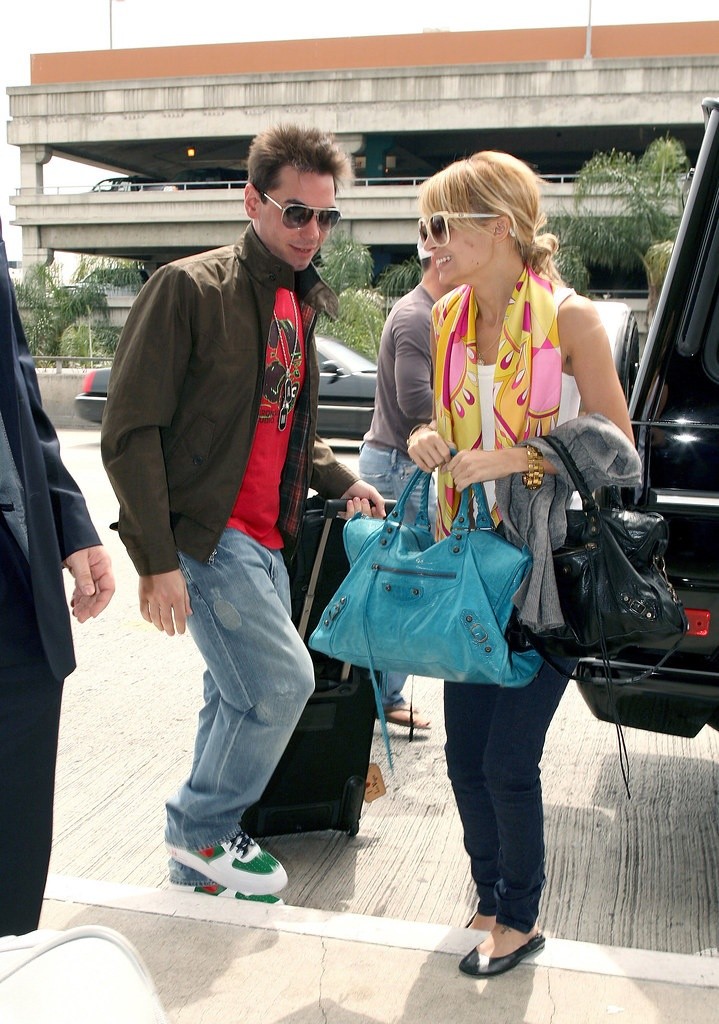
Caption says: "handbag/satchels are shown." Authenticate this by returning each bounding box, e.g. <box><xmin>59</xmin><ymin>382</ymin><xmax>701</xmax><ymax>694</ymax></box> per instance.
<box><xmin>308</xmin><ymin>447</ymin><xmax>542</xmax><ymax>690</ymax></box>
<box><xmin>507</xmin><ymin>433</ymin><xmax>688</xmax><ymax>685</ymax></box>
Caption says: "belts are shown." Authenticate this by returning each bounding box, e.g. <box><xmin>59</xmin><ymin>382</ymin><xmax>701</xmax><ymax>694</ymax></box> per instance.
<box><xmin>366</xmin><ymin>439</ymin><xmax>403</xmax><ymax>455</ymax></box>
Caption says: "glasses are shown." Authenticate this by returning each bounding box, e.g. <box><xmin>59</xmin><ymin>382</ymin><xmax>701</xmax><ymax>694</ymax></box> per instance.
<box><xmin>418</xmin><ymin>211</ymin><xmax>518</xmax><ymax>248</ymax></box>
<box><xmin>255</xmin><ymin>188</ymin><xmax>342</xmax><ymax>231</ymax></box>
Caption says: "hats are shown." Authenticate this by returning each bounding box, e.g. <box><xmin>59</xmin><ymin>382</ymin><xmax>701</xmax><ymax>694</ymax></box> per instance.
<box><xmin>417</xmin><ymin>240</ymin><xmax>432</xmax><ymax>259</ymax></box>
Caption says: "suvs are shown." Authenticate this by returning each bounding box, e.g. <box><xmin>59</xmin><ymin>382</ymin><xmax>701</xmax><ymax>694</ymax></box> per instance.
<box><xmin>533</xmin><ymin>100</ymin><xmax>719</xmax><ymax>741</ymax></box>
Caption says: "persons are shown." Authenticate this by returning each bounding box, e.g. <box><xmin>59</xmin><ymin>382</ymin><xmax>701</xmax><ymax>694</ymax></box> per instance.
<box><xmin>0</xmin><ymin>239</ymin><xmax>115</xmax><ymax>936</ymax></box>
<box><xmin>407</xmin><ymin>151</ymin><xmax>634</xmax><ymax>977</ymax></box>
<box><xmin>358</xmin><ymin>239</ymin><xmax>457</xmax><ymax>730</ymax></box>
<box><xmin>101</xmin><ymin>129</ymin><xmax>388</xmax><ymax>909</ymax></box>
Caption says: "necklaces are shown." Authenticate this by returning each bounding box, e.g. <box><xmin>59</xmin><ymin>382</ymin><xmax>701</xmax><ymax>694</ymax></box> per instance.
<box><xmin>476</xmin><ymin>334</ymin><xmax>500</xmax><ymax>365</ymax></box>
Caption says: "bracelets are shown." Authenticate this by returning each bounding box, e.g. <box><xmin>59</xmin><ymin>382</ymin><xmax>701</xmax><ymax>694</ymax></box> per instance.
<box><xmin>522</xmin><ymin>445</ymin><xmax>544</xmax><ymax>489</ymax></box>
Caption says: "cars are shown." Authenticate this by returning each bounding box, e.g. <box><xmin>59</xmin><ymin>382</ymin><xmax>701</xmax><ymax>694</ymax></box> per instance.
<box><xmin>75</xmin><ymin>333</ymin><xmax>378</xmax><ymax>442</ymax></box>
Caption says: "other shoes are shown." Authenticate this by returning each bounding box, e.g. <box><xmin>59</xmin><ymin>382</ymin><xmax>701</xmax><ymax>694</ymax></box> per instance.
<box><xmin>459</xmin><ymin>933</ymin><xmax>547</xmax><ymax>977</ymax></box>
<box><xmin>462</xmin><ymin>912</ymin><xmax>477</xmax><ymax>929</ymax></box>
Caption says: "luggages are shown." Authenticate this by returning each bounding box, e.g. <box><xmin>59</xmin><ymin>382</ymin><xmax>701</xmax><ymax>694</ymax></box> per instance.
<box><xmin>240</xmin><ymin>498</ymin><xmax>398</xmax><ymax>836</ymax></box>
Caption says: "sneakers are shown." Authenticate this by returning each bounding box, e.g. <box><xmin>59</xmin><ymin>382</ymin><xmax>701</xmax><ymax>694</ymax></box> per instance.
<box><xmin>168</xmin><ymin>833</ymin><xmax>288</xmax><ymax>895</ymax></box>
<box><xmin>192</xmin><ymin>886</ymin><xmax>284</xmax><ymax>905</ymax></box>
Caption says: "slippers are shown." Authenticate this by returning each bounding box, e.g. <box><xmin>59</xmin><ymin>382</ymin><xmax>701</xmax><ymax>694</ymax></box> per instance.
<box><xmin>378</xmin><ymin>699</ymin><xmax>432</xmax><ymax>730</ymax></box>
